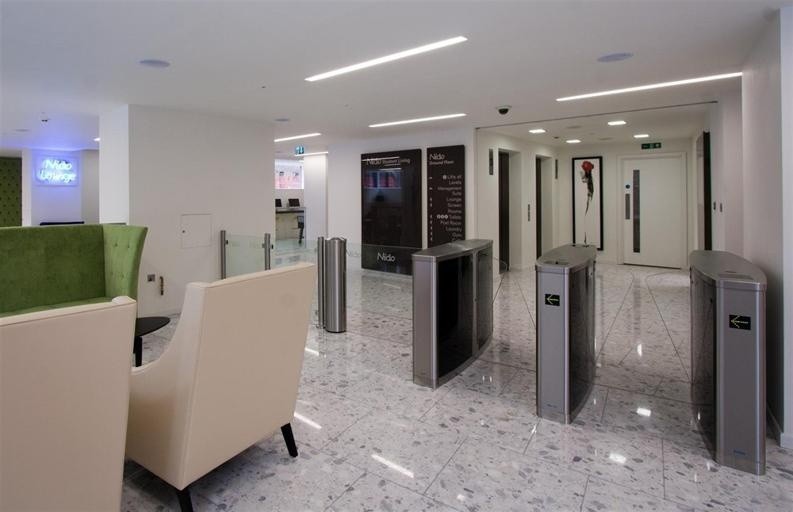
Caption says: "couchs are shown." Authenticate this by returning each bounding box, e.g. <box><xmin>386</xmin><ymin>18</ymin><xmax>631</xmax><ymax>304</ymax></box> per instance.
<box><xmin>0</xmin><ymin>222</ymin><xmax>148</xmax><ymax>318</ymax></box>
<box><xmin>0</xmin><ymin>295</ymin><xmax>138</xmax><ymax>507</ymax></box>
<box><xmin>125</xmin><ymin>261</ymin><xmax>317</xmax><ymax>511</ymax></box>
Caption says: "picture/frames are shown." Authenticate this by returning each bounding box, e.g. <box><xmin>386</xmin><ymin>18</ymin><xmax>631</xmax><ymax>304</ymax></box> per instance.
<box><xmin>571</xmin><ymin>155</ymin><xmax>603</xmax><ymax>251</ymax></box>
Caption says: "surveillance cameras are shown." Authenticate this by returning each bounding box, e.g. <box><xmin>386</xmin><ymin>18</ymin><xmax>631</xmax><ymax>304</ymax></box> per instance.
<box><xmin>497</xmin><ymin>105</ymin><xmax>512</xmax><ymax>114</ymax></box>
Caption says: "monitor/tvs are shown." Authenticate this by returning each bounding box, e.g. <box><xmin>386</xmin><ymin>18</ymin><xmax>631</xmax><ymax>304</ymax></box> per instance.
<box><xmin>363</xmin><ymin>167</ymin><xmax>407</xmax><ymax>208</ymax></box>
<box><xmin>275</xmin><ymin>200</ymin><xmax>282</xmax><ymax>207</ymax></box>
<box><xmin>288</xmin><ymin>199</ymin><xmax>299</xmax><ymax>208</ymax></box>
